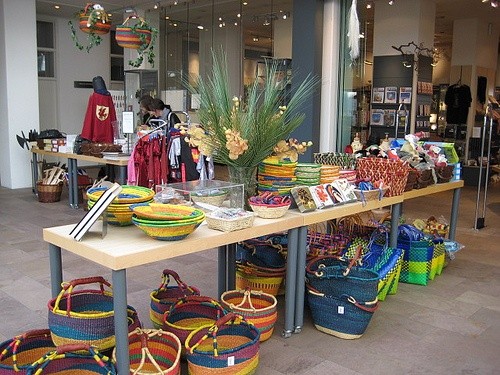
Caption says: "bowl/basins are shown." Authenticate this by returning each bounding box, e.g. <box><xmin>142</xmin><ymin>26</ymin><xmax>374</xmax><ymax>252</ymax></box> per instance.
<box><xmin>86</xmin><ymin>185</ymin><xmax>155</xmax><ymax>227</ymax></box>
<box><xmin>247</xmin><ymin>200</ymin><xmax>292</xmax><ymax>219</ymax></box>
<box><xmin>257</xmin><ymin>161</ymin><xmax>297</xmax><ymax>195</ymax></box>
<box><xmin>129</xmin><ymin>203</ymin><xmax>206</xmax><ymax>242</ymax></box>
<box><xmin>295</xmin><ymin>162</ymin><xmax>357</xmax><ymax>188</ymax></box>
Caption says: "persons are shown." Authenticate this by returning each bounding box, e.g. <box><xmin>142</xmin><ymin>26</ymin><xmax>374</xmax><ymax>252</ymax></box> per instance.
<box><xmin>134</xmin><ymin>89</ymin><xmax>200</xmax><ymax>183</ymax></box>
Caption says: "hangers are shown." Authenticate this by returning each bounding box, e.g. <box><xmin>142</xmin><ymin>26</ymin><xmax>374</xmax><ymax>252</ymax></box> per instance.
<box><xmin>452</xmin><ymin>83</ymin><xmax>462</xmax><ymax>86</ymax></box>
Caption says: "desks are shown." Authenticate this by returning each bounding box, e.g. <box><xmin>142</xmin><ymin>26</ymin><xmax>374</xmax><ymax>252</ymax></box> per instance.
<box><xmin>228</xmin><ymin>195</ymin><xmax>403</xmax><ymax>333</ymax></box>
<box><xmin>43</xmin><ymin>212</ymin><xmax>304</xmax><ymax>375</ymax></box>
<box><xmin>31</xmin><ymin>148</ymin><xmax>131</xmax><ymax>208</ymax></box>
<box><xmin>332</xmin><ymin>180</ymin><xmax>464</xmax><ymax>242</ymax></box>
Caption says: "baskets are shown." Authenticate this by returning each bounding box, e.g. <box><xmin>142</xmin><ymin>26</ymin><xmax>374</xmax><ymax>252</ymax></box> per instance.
<box><xmin>115</xmin><ymin>15</ymin><xmax>152</xmax><ymax>50</ymax></box>
<box><xmin>206</xmin><ymin>209</ymin><xmax>252</xmax><ymax>231</ymax></box>
<box><xmin>248</xmin><ymin>195</ymin><xmax>290</xmax><ymax>218</ymax></box>
<box><xmin>1</xmin><ymin>269</ymin><xmax>278</xmax><ymax>375</ymax></box>
<box><xmin>79</xmin><ymin>3</ymin><xmax>111</xmax><ymax>35</ymax></box>
<box><xmin>37</xmin><ymin>180</ymin><xmax>61</xmax><ymax>202</ymax></box>
<box><xmin>84</xmin><ymin>143</ymin><xmax>122</xmax><ymax>156</ymax></box>
<box><xmin>235</xmin><ymin>210</ymin><xmax>443</xmax><ymax>340</ymax></box>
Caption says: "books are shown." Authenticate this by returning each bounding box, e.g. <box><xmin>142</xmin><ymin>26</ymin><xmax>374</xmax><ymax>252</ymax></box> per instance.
<box><xmin>68</xmin><ymin>183</ymin><xmax>122</xmax><ymax>242</ymax></box>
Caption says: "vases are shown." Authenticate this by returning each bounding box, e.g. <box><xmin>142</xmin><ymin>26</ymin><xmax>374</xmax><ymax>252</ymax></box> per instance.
<box><xmin>228</xmin><ymin>166</ymin><xmax>258</xmax><ymax>211</ymax></box>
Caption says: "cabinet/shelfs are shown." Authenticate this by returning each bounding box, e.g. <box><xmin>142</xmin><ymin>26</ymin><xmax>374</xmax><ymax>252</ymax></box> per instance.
<box><xmin>371</xmin><ymin>103</ymin><xmax>410</xmax><ymax>130</ymax></box>
<box><xmin>416</xmin><ymin>93</ymin><xmax>433</xmax><ymax>129</ymax></box>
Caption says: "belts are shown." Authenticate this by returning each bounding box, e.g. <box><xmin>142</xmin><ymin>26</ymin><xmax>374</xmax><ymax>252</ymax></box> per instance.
<box><xmin>360</xmin><ymin>156</ymin><xmax>439</xmax><ymax>196</ymax></box>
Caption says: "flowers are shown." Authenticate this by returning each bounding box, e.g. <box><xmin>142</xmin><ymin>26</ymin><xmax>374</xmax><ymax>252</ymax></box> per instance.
<box><xmin>178</xmin><ymin>44</ymin><xmax>322</xmax><ymax>166</ymax></box>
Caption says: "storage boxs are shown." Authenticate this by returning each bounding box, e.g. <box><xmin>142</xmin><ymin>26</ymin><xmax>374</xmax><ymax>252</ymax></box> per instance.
<box><xmin>156</xmin><ymin>179</ymin><xmax>244</xmax><ymax>210</ymax></box>
